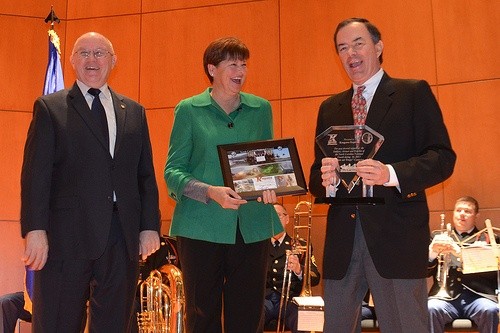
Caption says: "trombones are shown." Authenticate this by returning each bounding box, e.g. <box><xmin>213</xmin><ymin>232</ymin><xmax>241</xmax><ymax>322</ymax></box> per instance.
<box><xmin>277</xmin><ymin>192</ymin><xmax>313</xmax><ymax>333</ymax></box>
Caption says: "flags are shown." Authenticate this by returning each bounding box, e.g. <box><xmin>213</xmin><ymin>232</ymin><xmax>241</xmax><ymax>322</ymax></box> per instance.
<box><xmin>24</xmin><ymin>33</ymin><xmax>64</xmax><ymax>303</ymax></box>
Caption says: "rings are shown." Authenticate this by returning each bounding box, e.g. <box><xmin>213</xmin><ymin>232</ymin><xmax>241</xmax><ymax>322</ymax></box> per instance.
<box><xmin>153</xmin><ymin>248</ymin><xmax>156</xmax><ymax>251</ymax></box>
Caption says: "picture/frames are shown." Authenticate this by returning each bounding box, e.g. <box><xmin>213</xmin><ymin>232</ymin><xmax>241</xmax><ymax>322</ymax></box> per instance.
<box><xmin>217</xmin><ymin>137</ymin><xmax>308</xmax><ymax>200</ymax></box>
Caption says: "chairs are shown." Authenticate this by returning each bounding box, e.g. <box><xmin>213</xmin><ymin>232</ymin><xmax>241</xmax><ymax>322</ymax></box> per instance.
<box><xmin>360</xmin><ymin>291</ymin><xmax>499</xmax><ymax>333</ymax></box>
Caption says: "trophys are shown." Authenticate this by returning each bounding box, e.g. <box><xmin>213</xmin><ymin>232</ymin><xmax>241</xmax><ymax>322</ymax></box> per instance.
<box><xmin>313</xmin><ymin>125</ymin><xmax>385</xmax><ymax>205</ymax></box>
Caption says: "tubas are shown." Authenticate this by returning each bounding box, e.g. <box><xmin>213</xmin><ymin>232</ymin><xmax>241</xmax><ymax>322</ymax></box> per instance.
<box><xmin>136</xmin><ymin>264</ymin><xmax>188</xmax><ymax>333</ymax></box>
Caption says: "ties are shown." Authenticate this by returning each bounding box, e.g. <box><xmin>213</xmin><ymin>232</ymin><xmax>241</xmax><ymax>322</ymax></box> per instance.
<box><xmin>87</xmin><ymin>87</ymin><xmax>111</xmax><ymax>150</ymax></box>
<box><xmin>274</xmin><ymin>241</ymin><xmax>281</xmax><ymax>250</ymax></box>
<box><xmin>460</xmin><ymin>233</ymin><xmax>471</xmax><ymax>246</ymax></box>
<box><xmin>350</xmin><ymin>85</ymin><xmax>371</xmax><ymax>144</ymax></box>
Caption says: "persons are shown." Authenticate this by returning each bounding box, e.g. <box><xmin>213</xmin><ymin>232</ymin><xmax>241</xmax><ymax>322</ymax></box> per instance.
<box><xmin>163</xmin><ymin>37</ymin><xmax>284</xmax><ymax>333</ymax></box>
<box><xmin>427</xmin><ymin>196</ymin><xmax>500</xmax><ymax>333</ymax></box>
<box><xmin>264</xmin><ymin>202</ymin><xmax>321</xmax><ymax>333</ymax></box>
<box><xmin>309</xmin><ymin>18</ymin><xmax>456</xmax><ymax>333</ymax></box>
<box><xmin>19</xmin><ymin>31</ymin><xmax>162</xmax><ymax>333</ymax></box>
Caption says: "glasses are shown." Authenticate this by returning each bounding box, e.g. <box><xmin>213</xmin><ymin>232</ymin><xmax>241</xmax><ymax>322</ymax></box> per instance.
<box><xmin>73</xmin><ymin>48</ymin><xmax>114</xmax><ymax>59</ymax></box>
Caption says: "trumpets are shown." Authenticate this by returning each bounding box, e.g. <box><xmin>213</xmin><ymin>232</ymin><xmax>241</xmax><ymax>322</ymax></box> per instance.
<box><xmin>435</xmin><ymin>213</ymin><xmax>452</xmax><ymax>299</ymax></box>
